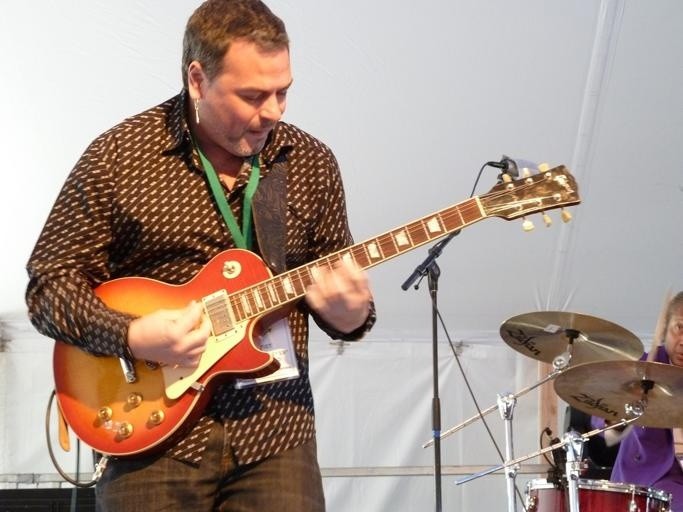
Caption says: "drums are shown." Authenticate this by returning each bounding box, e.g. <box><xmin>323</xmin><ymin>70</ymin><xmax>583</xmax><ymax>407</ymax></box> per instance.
<box><xmin>524</xmin><ymin>479</ymin><xmax>674</xmax><ymax>512</ymax></box>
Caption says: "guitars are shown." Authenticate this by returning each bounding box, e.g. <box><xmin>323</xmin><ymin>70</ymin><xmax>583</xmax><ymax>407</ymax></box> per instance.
<box><xmin>53</xmin><ymin>162</ymin><xmax>581</xmax><ymax>457</ymax></box>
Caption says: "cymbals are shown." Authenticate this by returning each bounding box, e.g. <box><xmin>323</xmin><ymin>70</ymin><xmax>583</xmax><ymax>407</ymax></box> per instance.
<box><xmin>500</xmin><ymin>311</ymin><xmax>644</xmax><ymax>366</ymax></box>
<box><xmin>554</xmin><ymin>359</ymin><xmax>683</xmax><ymax>428</ymax></box>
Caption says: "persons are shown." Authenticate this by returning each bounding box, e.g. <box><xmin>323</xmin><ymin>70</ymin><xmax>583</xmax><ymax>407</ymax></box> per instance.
<box><xmin>23</xmin><ymin>2</ymin><xmax>379</xmax><ymax>512</ymax></box>
<box><xmin>589</xmin><ymin>290</ymin><xmax>682</xmax><ymax>511</ymax></box>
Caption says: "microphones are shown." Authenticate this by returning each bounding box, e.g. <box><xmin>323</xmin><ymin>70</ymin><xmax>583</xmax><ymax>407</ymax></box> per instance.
<box><xmin>546</xmin><ymin>428</ymin><xmax>567</xmax><ymax>462</ymax></box>
<box><xmin>487</xmin><ymin>155</ymin><xmax>549</xmax><ymax>181</ymax></box>
